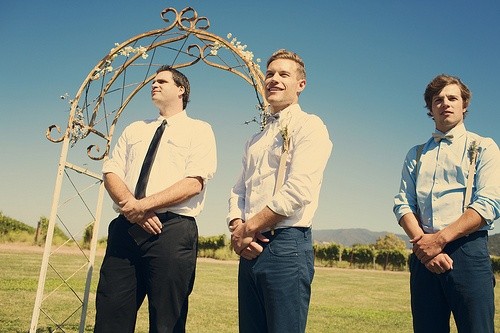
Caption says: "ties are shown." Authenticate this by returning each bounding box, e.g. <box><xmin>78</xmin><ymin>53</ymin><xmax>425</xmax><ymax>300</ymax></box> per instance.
<box><xmin>135</xmin><ymin>120</ymin><xmax>167</xmax><ymax>200</ymax></box>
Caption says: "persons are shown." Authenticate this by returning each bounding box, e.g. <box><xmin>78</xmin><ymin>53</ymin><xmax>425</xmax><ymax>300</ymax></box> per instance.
<box><xmin>392</xmin><ymin>80</ymin><xmax>500</xmax><ymax>333</ymax></box>
<box><xmin>226</xmin><ymin>47</ymin><xmax>333</xmax><ymax>333</ymax></box>
<box><xmin>93</xmin><ymin>64</ymin><xmax>217</xmax><ymax>333</ymax></box>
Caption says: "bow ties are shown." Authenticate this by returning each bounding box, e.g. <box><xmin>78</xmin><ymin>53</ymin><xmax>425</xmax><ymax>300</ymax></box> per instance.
<box><xmin>266</xmin><ymin>112</ymin><xmax>282</xmax><ymax>122</ymax></box>
<box><xmin>432</xmin><ymin>132</ymin><xmax>454</xmax><ymax>144</ymax></box>
